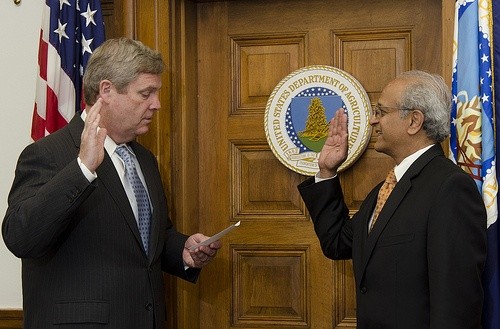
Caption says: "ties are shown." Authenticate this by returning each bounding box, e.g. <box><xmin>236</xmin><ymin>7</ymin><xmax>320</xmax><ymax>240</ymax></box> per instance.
<box><xmin>115</xmin><ymin>146</ymin><xmax>151</xmax><ymax>256</ymax></box>
<box><xmin>370</xmin><ymin>169</ymin><xmax>398</xmax><ymax>232</ymax></box>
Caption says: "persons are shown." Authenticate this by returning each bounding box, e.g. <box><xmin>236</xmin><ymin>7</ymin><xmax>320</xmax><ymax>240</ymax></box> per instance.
<box><xmin>297</xmin><ymin>70</ymin><xmax>488</xmax><ymax>329</ymax></box>
<box><xmin>1</xmin><ymin>38</ymin><xmax>222</xmax><ymax>329</ymax></box>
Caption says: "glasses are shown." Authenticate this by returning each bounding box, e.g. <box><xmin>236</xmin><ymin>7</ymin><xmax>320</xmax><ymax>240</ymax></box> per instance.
<box><xmin>372</xmin><ymin>105</ymin><xmax>426</xmax><ymax>122</ymax></box>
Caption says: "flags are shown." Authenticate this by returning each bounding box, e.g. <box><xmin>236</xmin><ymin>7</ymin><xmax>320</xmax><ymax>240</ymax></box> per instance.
<box><xmin>31</xmin><ymin>0</ymin><xmax>106</xmax><ymax>141</ymax></box>
<box><xmin>449</xmin><ymin>0</ymin><xmax>500</xmax><ymax>329</ymax></box>
